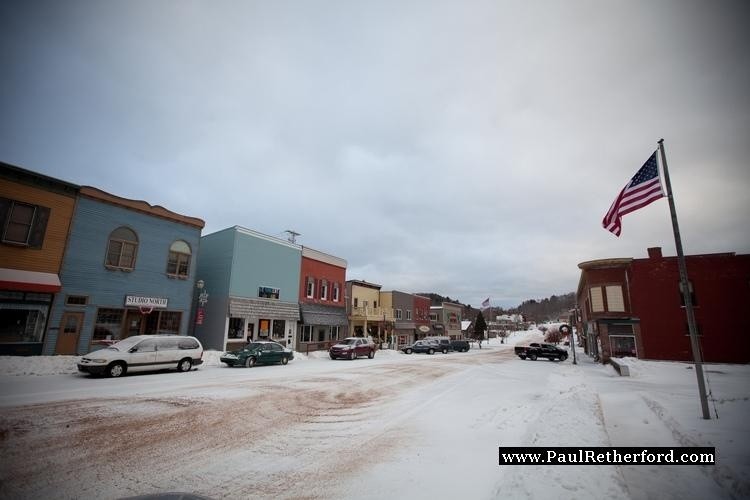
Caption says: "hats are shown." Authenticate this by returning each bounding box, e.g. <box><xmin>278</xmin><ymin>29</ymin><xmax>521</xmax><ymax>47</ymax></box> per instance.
<box><xmin>451</xmin><ymin>340</ymin><xmax>470</xmax><ymax>352</ymax></box>
<box><xmin>401</xmin><ymin>340</ymin><xmax>439</xmax><ymax>354</ymax></box>
<box><xmin>219</xmin><ymin>341</ymin><xmax>294</xmax><ymax>368</ymax></box>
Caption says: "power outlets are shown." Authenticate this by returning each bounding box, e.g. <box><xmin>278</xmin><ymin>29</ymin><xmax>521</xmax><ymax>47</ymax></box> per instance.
<box><xmin>514</xmin><ymin>342</ymin><xmax>568</xmax><ymax>361</ymax></box>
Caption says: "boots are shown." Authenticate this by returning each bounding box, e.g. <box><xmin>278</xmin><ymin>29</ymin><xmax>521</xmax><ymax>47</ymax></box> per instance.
<box><xmin>329</xmin><ymin>337</ymin><xmax>378</xmax><ymax>360</ymax></box>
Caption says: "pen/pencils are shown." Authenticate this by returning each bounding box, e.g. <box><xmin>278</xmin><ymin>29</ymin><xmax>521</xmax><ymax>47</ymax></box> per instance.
<box><xmin>423</xmin><ymin>336</ymin><xmax>454</xmax><ymax>354</ymax></box>
<box><xmin>77</xmin><ymin>334</ymin><xmax>204</xmax><ymax>379</ymax></box>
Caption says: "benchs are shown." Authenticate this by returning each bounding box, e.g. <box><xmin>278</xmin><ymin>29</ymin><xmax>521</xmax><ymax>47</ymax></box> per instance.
<box><xmin>482</xmin><ymin>298</ymin><xmax>489</xmax><ymax>307</ymax></box>
<box><xmin>601</xmin><ymin>150</ymin><xmax>666</xmax><ymax>238</ymax></box>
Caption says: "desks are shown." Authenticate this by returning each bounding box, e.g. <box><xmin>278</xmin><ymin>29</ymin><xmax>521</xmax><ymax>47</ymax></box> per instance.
<box><xmin>192</xmin><ymin>278</ymin><xmax>206</xmax><ymax>335</ymax></box>
